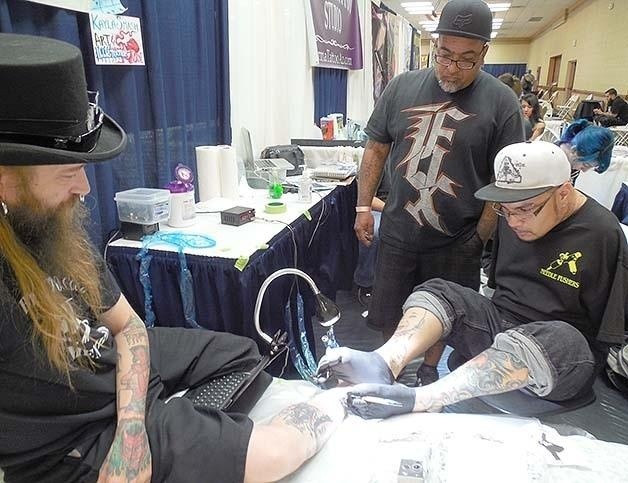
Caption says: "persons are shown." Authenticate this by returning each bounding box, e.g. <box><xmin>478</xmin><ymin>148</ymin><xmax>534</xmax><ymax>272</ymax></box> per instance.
<box><xmin>496</xmin><ymin>68</ymin><xmax>628</xmax><ymax>188</ymax></box>
<box><xmin>352</xmin><ymin>0</ymin><xmax>527</xmax><ymax>388</ymax></box>
<box><xmin>309</xmin><ymin>139</ymin><xmax>628</xmax><ymax>423</ymax></box>
<box><xmin>353</xmin><ymin>156</ymin><xmax>392</xmax><ymax>307</ymax></box>
<box><xmin>0</xmin><ymin>30</ymin><xmax>348</xmax><ymax>482</ymax></box>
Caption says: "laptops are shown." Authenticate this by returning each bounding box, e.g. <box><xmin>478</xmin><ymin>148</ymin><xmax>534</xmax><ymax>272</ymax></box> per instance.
<box><xmin>181</xmin><ymin>354</ymin><xmax>271</xmax><ymax>413</ymax></box>
<box><xmin>241</xmin><ymin>126</ymin><xmax>296</xmax><ymax>171</ymax></box>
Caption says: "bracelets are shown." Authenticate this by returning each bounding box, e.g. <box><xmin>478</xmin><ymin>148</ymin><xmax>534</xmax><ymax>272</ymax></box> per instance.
<box><xmin>355</xmin><ymin>206</ymin><xmax>372</xmax><ymax>213</ymax></box>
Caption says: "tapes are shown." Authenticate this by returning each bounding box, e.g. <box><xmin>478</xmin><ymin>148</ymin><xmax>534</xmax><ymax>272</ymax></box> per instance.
<box><xmin>265</xmin><ymin>201</ymin><xmax>286</xmax><ymax>213</ymax></box>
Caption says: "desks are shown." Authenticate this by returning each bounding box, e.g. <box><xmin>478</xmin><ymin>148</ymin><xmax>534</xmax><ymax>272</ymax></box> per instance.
<box><xmin>108</xmin><ymin>134</ymin><xmax>367</xmax><ymax>377</ymax></box>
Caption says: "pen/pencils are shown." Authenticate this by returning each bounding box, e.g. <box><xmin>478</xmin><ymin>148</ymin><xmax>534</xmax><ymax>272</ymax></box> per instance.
<box><xmin>351</xmin><ymin>394</ymin><xmax>404</xmax><ymax>409</ymax></box>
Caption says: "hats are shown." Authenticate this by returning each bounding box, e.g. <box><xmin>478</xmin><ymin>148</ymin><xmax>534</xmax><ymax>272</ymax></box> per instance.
<box><xmin>1</xmin><ymin>32</ymin><xmax>128</xmax><ymax>166</ymax></box>
<box><xmin>430</xmin><ymin>0</ymin><xmax>493</xmax><ymax>42</ymax></box>
<box><xmin>475</xmin><ymin>140</ymin><xmax>571</xmax><ymax>204</ymax></box>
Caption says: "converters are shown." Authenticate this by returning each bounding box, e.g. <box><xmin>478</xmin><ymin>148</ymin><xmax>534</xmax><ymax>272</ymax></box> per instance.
<box><xmin>220</xmin><ymin>206</ymin><xmax>256</xmax><ymax>226</ymax></box>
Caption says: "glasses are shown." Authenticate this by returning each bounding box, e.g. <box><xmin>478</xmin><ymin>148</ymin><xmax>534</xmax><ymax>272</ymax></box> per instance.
<box><xmin>433</xmin><ymin>38</ymin><xmax>485</xmax><ymax>70</ymax></box>
<box><xmin>491</xmin><ymin>187</ymin><xmax>560</xmax><ymax>222</ymax></box>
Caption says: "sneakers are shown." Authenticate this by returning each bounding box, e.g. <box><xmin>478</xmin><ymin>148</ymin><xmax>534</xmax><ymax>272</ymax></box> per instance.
<box><xmin>415</xmin><ymin>372</ymin><xmax>442</xmax><ymax>413</ymax></box>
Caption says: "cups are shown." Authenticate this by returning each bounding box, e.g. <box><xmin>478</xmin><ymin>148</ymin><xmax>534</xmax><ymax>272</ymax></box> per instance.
<box><xmin>320</xmin><ymin>118</ymin><xmax>334</xmax><ymax>140</ymax></box>
<box><xmin>269</xmin><ymin>168</ymin><xmax>284</xmax><ymax>199</ymax></box>
<box><xmin>298</xmin><ymin>178</ymin><xmax>313</xmax><ymax>203</ymax></box>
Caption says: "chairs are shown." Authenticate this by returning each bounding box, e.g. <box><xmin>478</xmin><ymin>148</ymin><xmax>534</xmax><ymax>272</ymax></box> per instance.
<box><xmin>535</xmin><ymin>90</ymin><xmax>592</xmax><ymax>138</ymax></box>
<box><xmin>606</xmin><ymin>124</ymin><xmax>628</xmax><ymax>147</ymax></box>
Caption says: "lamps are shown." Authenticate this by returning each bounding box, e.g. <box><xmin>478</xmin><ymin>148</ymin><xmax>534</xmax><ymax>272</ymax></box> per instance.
<box><xmin>253</xmin><ymin>267</ymin><xmax>340</xmax><ymax>353</ymax></box>
